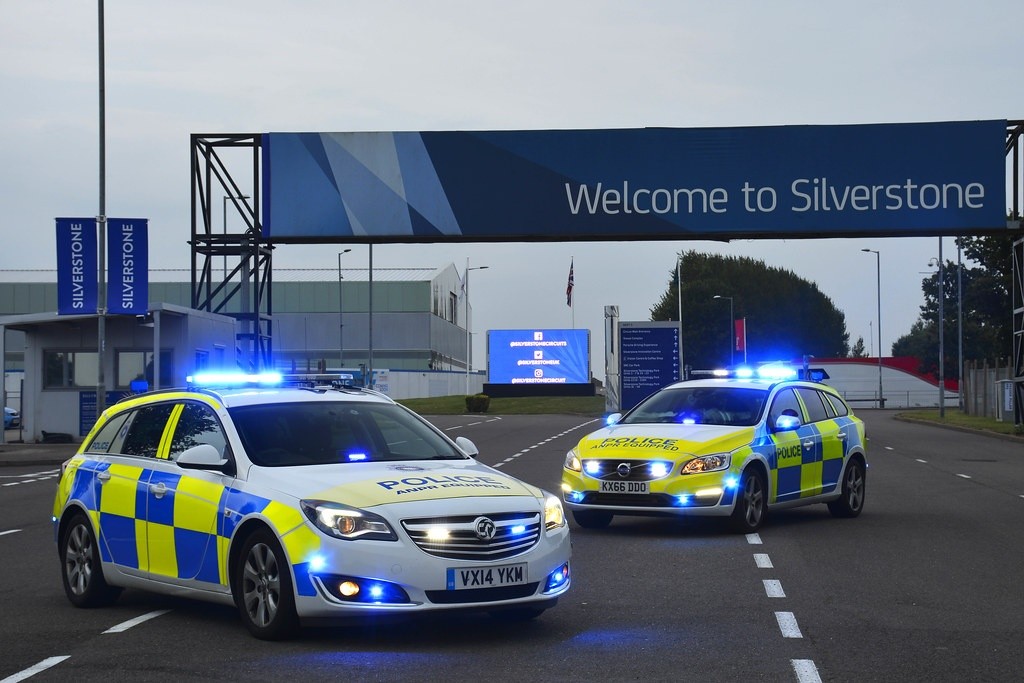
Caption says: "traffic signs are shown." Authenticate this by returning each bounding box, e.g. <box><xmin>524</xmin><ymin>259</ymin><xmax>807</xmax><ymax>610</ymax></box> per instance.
<box><xmin>617</xmin><ymin>321</ymin><xmax>683</xmax><ymax>411</ymax></box>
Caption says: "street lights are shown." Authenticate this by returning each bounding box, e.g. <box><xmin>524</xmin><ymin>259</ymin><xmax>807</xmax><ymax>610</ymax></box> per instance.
<box><xmin>713</xmin><ymin>296</ymin><xmax>734</xmax><ymax>370</ymax></box>
<box><xmin>223</xmin><ymin>195</ymin><xmax>252</xmax><ymax>313</ymax></box>
<box><xmin>860</xmin><ymin>248</ymin><xmax>885</xmax><ymax>408</ymax></box>
<box><xmin>466</xmin><ymin>266</ymin><xmax>491</xmax><ymax>396</ymax></box>
<box><xmin>927</xmin><ymin>236</ymin><xmax>944</xmax><ymax>415</ymax></box>
<box><xmin>337</xmin><ymin>249</ymin><xmax>353</xmax><ymax>368</ymax></box>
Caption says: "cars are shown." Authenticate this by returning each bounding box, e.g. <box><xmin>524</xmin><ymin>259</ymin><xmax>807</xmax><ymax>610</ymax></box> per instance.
<box><xmin>562</xmin><ymin>363</ymin><xmax>869</xmax><ymax>534</ymax></box>
<box><xmin>53</xmin><ymin>371</ymin><xmax>574</xmax><ymax>641</ymax></box>
<box><xmin>4</xmin><ymin>407</ymin><xmax>20</xmax><ymax>428</ymax></box>
